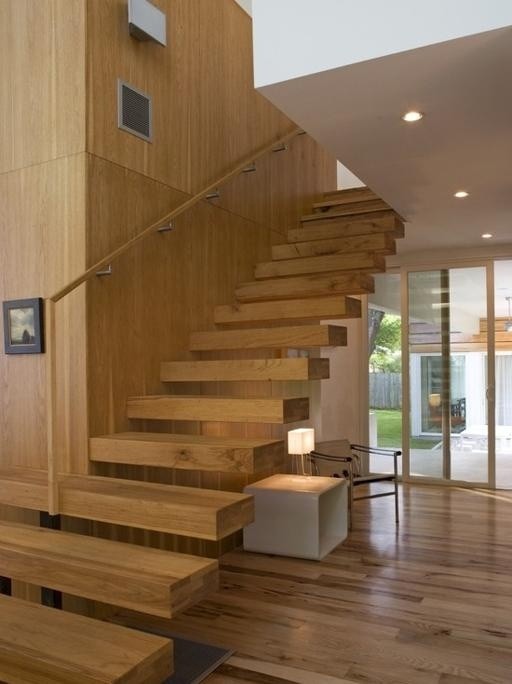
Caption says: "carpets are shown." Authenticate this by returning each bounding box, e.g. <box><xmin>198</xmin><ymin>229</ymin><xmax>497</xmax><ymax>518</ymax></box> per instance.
<box><xmin>126</xmin><ymin>623</ymin><xmax>239</xmax><ymax>684</ymax></box>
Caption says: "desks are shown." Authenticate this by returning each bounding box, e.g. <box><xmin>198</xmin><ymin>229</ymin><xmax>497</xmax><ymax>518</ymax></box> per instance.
<box><xmin>243</xmin><ymin>473</ymin><xmax>349</xmax><ymax>561</ymax></box>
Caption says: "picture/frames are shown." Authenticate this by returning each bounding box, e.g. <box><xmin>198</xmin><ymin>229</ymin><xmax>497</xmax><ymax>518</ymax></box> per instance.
<box><xmin>2</xmin><ymin>296</ymin><xmax>44</xmax><ymax>354</ymax></box>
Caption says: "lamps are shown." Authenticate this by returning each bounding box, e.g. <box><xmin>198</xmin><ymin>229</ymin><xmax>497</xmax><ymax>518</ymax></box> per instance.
<box><xmin>287</xmin><ymin>427</ymin><xmax>316</xmax><ymax>476</ymax></box>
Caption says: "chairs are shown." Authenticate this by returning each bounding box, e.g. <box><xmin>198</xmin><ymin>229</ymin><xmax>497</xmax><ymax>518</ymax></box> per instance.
<box><xmin>305</xmin><ymin>439</ymin><xmax>402</xmax><ymax>530</ymax></box>
